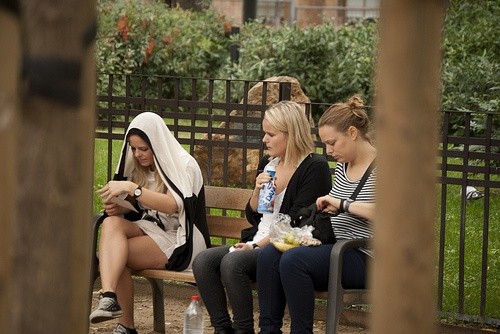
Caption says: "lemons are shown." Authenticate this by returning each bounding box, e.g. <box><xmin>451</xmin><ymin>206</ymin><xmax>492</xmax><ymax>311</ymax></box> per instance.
<box><xmin>283</xmin><ymin>235</ymin><xmax>300</xmax><ymax>246</ymax></box>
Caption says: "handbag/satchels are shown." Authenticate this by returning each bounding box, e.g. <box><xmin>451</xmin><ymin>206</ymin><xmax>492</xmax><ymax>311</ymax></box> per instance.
<box><xmin>290</xmin><ymin>203</ymin><xmax>337</xmax><ymax>245</ymax></box>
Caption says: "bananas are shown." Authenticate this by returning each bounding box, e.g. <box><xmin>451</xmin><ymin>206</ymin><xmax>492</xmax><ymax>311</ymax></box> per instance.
<box><xmin>273</xmin><ymin>241</ymin><xmax>297</xmax><ymax>251</ymax></box>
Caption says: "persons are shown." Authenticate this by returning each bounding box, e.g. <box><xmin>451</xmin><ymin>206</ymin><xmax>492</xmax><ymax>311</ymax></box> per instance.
<box><xmin>256</xmin><ymin>95</ymin><xmax>376</xmax><ymax>334</ymax></box>
<box><xmin>89</xmin><ymin>112</ymin><xmax>211</xmax><ymax>334</ymax></box>
<box><xmin>192</xmin><ymin>100</ymin><xmax>333</xmax><ymax>334</ymax></box>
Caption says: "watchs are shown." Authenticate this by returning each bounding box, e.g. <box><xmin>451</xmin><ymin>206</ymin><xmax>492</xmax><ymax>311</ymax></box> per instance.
<box><xmin>252</xmin><ymin>244</ymin><xmax>260</xmax><ymax>249</ymax></box>
<box><xmin>344</xmin><ymin>199</ymin><xmax>354</xmax><ymax>213</ymax></box>
<box><xmin>132</xmin><ymin>186</ymin><xmax>142</xmax><ymax>198</ymax></box>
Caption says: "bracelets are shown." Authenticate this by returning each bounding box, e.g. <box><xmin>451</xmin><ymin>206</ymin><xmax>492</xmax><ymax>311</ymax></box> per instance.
<box><xmin>340</xmin><ymin>198</ymin><xmax>347</xmax><ymax>213</ymax></box>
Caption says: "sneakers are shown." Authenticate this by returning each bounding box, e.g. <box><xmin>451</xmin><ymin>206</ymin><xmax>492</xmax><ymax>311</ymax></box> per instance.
<box><xmin>89</xmin><ymin>289</ymin><xmax>123</xmax><ymax>323</ymax></box>
<box><xmin>110</xmin><ymin>323</ymin><xmax>138</xmax><ymax>334</ymax></box>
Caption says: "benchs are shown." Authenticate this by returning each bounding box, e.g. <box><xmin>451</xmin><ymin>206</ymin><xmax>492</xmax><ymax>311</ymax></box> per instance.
<box><xmin>93</xmin><ymin>187</ymin><xmax>372</xmax><ymax>334</ymax></box>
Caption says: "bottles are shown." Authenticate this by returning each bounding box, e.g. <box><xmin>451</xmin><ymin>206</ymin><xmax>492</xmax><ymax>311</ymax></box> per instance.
<box><xmin>257</xmin><ymin>165</ymin><xmax>277</xmax><ymax>214</ymax></box>
<box><xmin>184</xmin><ymin>295</ymin><xmax>205</xmax><ymax>334</ymax></box>
